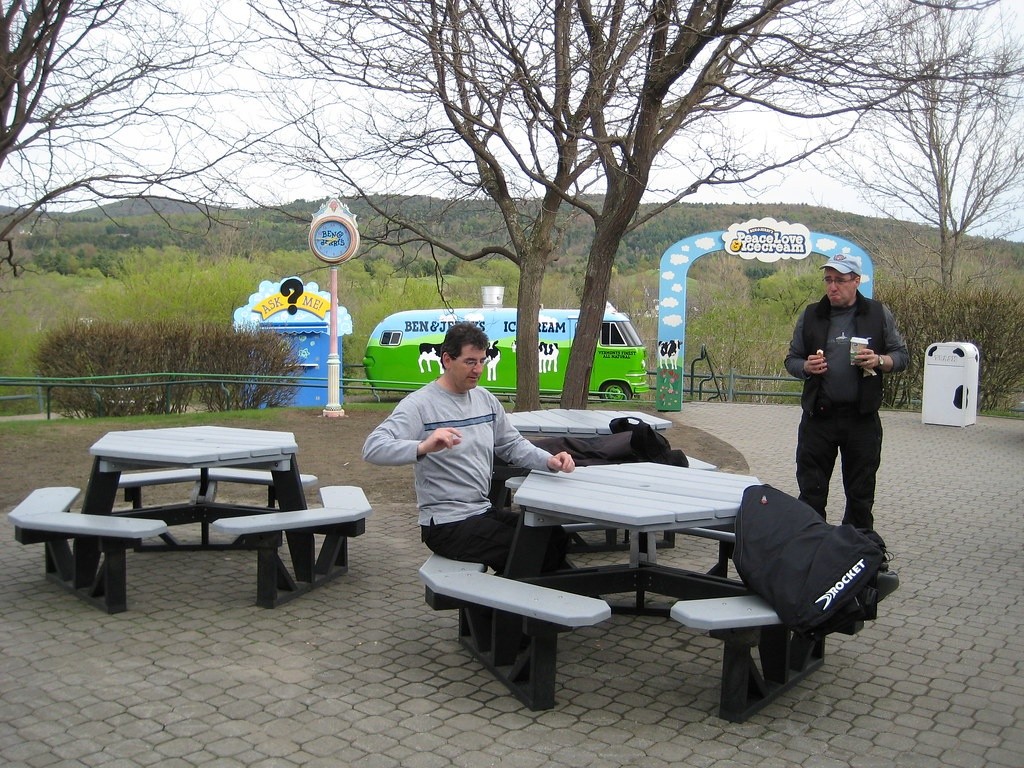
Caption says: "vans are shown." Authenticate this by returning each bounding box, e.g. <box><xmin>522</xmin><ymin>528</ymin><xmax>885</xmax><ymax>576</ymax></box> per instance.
<box><xmin>362</xmin><ymin>308</ymin><xmax>650</xmax><ymax>404</ymax></box>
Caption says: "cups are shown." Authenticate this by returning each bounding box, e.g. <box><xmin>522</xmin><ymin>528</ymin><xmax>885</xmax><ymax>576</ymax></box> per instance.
<box><xmin>850</xmin><ymin>337</ymin><xmax>869</xmax><ymax>365</ymax></box>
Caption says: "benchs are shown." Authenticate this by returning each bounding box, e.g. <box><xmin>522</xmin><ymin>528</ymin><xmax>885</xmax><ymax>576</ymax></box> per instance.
<box><xmin>7</xmin><ymin>408</ymin><xmax>902</xmax><ymax>725</ymax></box>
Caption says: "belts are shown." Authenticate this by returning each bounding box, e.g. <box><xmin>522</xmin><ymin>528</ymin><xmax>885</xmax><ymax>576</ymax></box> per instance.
<box><xmin>816</xmin><ymin>406</ymin><xmax>848</xmax><ymax>413</ymax></box>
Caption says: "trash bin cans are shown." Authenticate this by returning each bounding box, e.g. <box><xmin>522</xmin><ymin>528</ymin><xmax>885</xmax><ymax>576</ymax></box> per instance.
<box><xmin>919</xmin><ymin>341</ymin><xmax>981</xmax><ymax>426</ymax></box>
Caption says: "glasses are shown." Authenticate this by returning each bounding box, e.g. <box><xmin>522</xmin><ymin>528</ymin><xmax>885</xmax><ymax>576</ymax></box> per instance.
<box><xmin>453</xmin><ymin>356</ymin><xmax>487</xmax><ymax>368</ymax></box>
<box><xmin>822</xmin><ymin>276</ymin><xmax>854</xmax><ymax>285</ymax></box>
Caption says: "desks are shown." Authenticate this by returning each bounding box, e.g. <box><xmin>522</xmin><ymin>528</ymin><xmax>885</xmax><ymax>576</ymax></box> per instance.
<box><xmin>70</xmin><ymin>425</ymin><xmax>317</xmax><ymax>586</ymax></box>
<box><xmin>492</xmin><ymin>462</ymin><xmax>792</xmax><ymax>687</ymax></box>
<box><xmin>504</xmin><ymin>409</ymin><xmax>673</xmax><ymax>439</ymax></box>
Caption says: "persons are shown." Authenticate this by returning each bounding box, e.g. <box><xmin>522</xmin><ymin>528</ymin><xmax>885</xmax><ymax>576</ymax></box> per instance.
<box><xmin>362</xmin><ymin>321</ymin><xmax>575</xmax><ymax>632</ymax></box>
<box><xmin>784</xmin><ymin>253</ymin><xmax>910</xmax><ymax>571</ymax></box>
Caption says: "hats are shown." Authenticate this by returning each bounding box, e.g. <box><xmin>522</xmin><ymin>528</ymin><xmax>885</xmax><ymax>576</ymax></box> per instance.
<box><xmin>819</xmin><ymin>253</ymin><xmax>861</xmax><ymax>276</ymax></box>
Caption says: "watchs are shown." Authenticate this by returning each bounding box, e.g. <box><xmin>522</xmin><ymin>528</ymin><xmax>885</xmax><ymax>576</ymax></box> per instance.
<box><xmin>876</xmin><ymin>354</ymin><xmax>884</xmax><ymax>367</ymax></box>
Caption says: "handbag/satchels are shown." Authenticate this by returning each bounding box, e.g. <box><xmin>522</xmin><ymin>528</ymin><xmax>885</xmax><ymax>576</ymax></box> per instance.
<box><xmin>609</xmin><ymin>416</ymin><xmax>671</xmax><ymax>458</ymax></box>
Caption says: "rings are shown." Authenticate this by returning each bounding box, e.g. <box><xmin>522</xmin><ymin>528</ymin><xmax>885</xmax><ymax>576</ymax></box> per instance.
<box><xmin>867</xmin><ymin>361</ymin><xmax>869</xmax><ymax>365</ymax></box>
